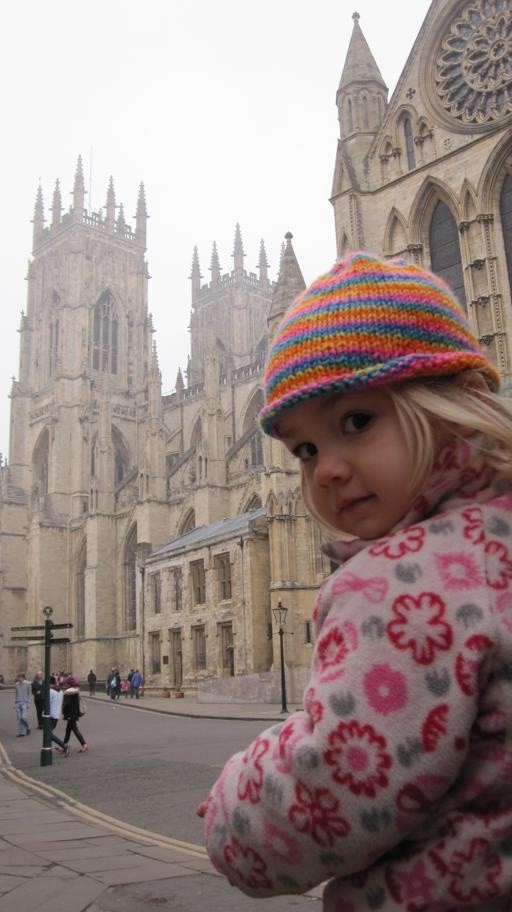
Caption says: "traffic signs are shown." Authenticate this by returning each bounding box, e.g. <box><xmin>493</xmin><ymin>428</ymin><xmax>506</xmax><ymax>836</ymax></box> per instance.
<box><xmin>10</xmin><ymin>625</ymin><xmax>44</xmax><ymax>632</ymax></box>
<box><xmin>50</xmin><ymin>638</ymin><xmax>71</xmax><ymax>644</ymax></box>
<box><xmin>10</xmin><ymin>636</ymin><xmax>45</xmax><ymax>642</ymax></box>
<box><xmin>50</xmin><ymin>622</ymin><xmax>76</xmax><ymax>630</ymax></box>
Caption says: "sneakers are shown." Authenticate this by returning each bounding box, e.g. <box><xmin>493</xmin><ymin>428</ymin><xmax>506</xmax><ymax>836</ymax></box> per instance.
<box><xmin>55</xmin><ymin>743</ymin><xmax>89</xmax><ymax>757</ymax></box>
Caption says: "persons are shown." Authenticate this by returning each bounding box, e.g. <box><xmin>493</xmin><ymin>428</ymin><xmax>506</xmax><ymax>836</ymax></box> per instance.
<box><xmin>13</xmin><ymin>667</ymin><xmax>143</xmax><ymax>757</ymax></box>
<box><xmin>196</xmin><ymin>251</ymin><xmax>511</xmax><ymax>911</ymax></box>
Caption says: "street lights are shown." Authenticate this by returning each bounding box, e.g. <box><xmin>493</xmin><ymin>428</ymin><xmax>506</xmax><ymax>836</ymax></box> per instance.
<box><xmin>271</xmin><ymin>597</ymin><xmax>290</xmax><ymax>715</ymax></box>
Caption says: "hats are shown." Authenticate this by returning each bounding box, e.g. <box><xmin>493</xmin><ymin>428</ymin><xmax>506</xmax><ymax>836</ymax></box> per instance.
<box><xmin>253</xmin><ymin>251</ymin><xmax>504</xmax><ymax>437</ymax></box>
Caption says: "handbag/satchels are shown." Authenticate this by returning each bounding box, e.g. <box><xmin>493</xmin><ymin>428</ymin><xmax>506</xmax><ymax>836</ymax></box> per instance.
<box><xmin>78</xmin><ymin>695</ymin><xmax>87</xmax><ymax>718</ymax></box>
